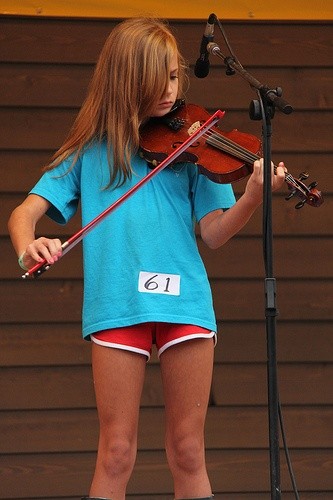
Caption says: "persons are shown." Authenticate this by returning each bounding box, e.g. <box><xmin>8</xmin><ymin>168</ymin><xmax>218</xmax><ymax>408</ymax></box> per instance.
<box><xmin>8</xmin><ymin>17</ymin><xmax>288</xmax><ymax>500</ymax></box>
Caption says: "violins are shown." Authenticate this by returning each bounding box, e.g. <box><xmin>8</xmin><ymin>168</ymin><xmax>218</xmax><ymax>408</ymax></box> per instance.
<box><xmin>136</xmin><ymin>103</ymin><xmax>323</xmax><ymax>212</ymax></box>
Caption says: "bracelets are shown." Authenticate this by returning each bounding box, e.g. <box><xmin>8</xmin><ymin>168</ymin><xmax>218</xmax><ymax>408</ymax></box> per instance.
<box><xmin>18</xmin><ymin>253</ymin><xmax>28</xmax><ymax>271</ymax></box>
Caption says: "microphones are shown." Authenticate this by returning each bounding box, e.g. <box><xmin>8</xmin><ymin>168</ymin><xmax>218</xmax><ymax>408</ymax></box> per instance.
<box><xmin>194</xmin><ymin>14</ymin><xmax>215</xmax><ymax>77</ymax></box>
